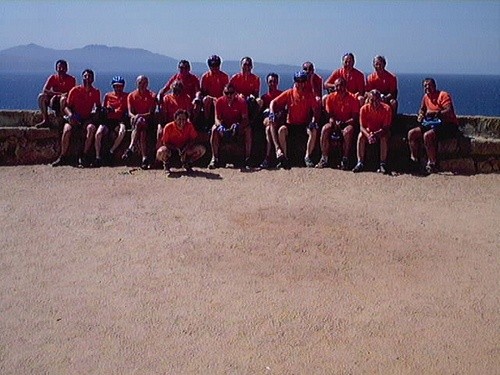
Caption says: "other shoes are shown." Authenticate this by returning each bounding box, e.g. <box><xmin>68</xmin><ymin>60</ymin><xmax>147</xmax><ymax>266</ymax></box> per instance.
<box><xmin>141</xmin><ymin>158</ymin><xmax>152</xmax><ymax>169</ymax></box>
<box><xmin>78</xmin><ymin>153</ymin><xmax>90</xmax><ymax>168</ymax></box>
<box><xmin>304</xmin><ymin>156</ymin><xmax>316</xmax><ymax>167</ymax></box>
<box><xmin>207</xmin><ymin>159</ymin><xmax>220</xmax><ymax>168</ymax></box>
<box><xmin>379</xmin><ymin>162</ymin><xmax>390</xmax><ymax>174</ymax></box>
<box><xmin>94</xmin><ymin>148</ymin><xmax>114</xmax><ymax>167</ymax></box>
<box><xmin>352</xmin><ymin>159</ymin><xmax>369</xmax><ymax>172</ymax></box>
<box><xmin>276</xmin><ymin>157</ymin><xmax>288</xmax><ymax>167</ymax></box>
<box><xmin>203</xmin><ymin>121</ymin><xmax>211</xmax><ymax>131</ymax></box>
<box><xmin>341</xmin><ymin>159</ymin><xmax>351</xmax><ymax>170</ymax></box>
<box><xmin>245</xmin><ymin>160</ymin><xmax>262</xmax><ymax>171</ymax></box>
<box><xmin>36</xmin><ymin>119</ymin><xmax>52</xmax><ymax>128</ymax></box>
<box><xmin>52</xmin><ymin>155</ymin><xmax>69</xmax><ymax>167</ymax></box>
<box><xmin>260</xmin><ymin>159</ymin><xmax>272</xmax><ymax>168</ymax></box>
<box><xmin>315</xmin><ymin>158</ymin><xmax>330</xmax><ymax>168</ymax></box>
<box><xmin>276</xmin><ymin>147</ymin><xmax>284</xmax><ymax>158</ymax></box>
<box><xmin>122</xmin><ymin>149</ymin><xmax>133</xmax><ymax>161</ymax></box>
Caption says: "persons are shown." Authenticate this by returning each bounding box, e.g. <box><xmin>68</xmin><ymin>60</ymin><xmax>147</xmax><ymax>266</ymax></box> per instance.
<box><xmin>89</xmin><ymin>75</ymin><xmax>130</xmax><ymax>167</ymax></box>
<box><xmin>403</xmin><ymin>77</ymin><xmax>459</xmax><ymax>177</ymax></box>
<box><xmin>35</xmin><ymin>59</ymin><xmax>76</xmax><ymax>129</ymax></box>
<box><xmin>155</xmin><ymin>53</ymin><xmax>400</xmax><ymax>174</ymax></box>
<box><xmin>52</xmin><ymin>69</ymin><xmax>101</xmax><ymax>168</ymax></box>
<box><xmin>121</xmin><ymin>74</ymin><xmax>157</xmax><ymax>170</ymax></box>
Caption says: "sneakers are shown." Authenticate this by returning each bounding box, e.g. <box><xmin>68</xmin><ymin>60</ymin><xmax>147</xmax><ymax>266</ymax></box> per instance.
<box><xmin>424</xmin><ymin>157</ymin><xmax>440</xmax><ymax>174</ymax></box>
<box><xmin>404</xmin><ymin>157</ymin><xmax>420</xmax><ymax>173</ymax></box>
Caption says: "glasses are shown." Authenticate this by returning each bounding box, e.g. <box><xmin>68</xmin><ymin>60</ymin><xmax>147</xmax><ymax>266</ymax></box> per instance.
<box><xmin>269</xmin><ymin>80</ymin><xmax>273</xmax><ymax>83</ymax></box>
<box><xmin>223</xmin><ymin>91</ymin><xmax>234</xmax><ymax>94</ymax></box>
<box><xmin>243</xmin><ymin>63</ymin><xmax>250</xmax><ymax>66</ymax></box>
<box><xmin>303</xmin><ymin>67</ymin><xmax>312</xmax><ymax>70</ymax></box>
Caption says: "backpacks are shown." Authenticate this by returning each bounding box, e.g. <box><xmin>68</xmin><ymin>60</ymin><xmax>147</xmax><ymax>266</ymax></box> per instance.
<box><xmin>420</xmin><ymin>114</ymin><xmax>445</xmax><ymax>132</ymax></box>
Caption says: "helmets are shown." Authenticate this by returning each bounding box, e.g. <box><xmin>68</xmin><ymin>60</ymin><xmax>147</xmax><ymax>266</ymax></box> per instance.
<box><xmin>208</xmin><ymin>55</ymin><xmax>220</xmax><ymax>67</ymax></box>
<box><xmin>295</xmin><ymin>70</ymin><xmax>307</xmax><ymax>82</ymax></box>
<box><xmin>112</xmin><ymin>76</ymin><xmax>125</xmax><ymax>86</ymax></box>
<box><xmin>330</xmin><ymin>134</ymin><xmax>343</xmax><ymax>146</ymax></box>
